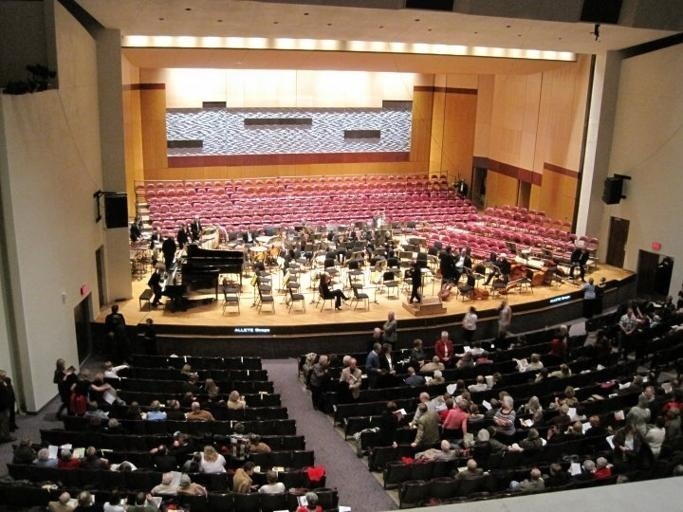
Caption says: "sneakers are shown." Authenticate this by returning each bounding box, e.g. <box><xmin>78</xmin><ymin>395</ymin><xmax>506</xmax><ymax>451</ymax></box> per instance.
<box><xmin>569</xmin><ymin>277</ymin><xmax>588</xmax><ymax>283</ymax></box>
<box><xmin>152</xmin><ymin>301</ymin><xmax>165</xmax><ymax>308</ymax></box>
<box><xmin>409</xmin><ymin>299</ymin><xmax>423</xmax><ymax>304</ymax></box>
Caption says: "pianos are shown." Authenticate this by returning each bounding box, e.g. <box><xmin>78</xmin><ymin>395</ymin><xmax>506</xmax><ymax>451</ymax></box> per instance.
<box><xmin>160</xmin><ymin>245</ymin><xmax>244</xmax><ymax>312</ymax></box>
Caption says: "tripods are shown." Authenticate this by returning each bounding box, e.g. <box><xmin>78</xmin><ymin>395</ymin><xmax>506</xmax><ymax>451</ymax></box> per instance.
<box><xmin>369</xmin><ymin>283</ymin><xmax>379</xmax><ymax>305</ymax></box>
<box><xmin>251</xmin><ymin>288</ymin><xmax>261</xmax><ymax>309</ymax></box>
<box><xmin>309</xmin><ymin>282</ymin><xmax>320</xmax><ymax>304</ymax></box>
<box><xmin>274</xmin><ymin>272</ymin><xmax>283</xmax><ymax>293</ymax></box>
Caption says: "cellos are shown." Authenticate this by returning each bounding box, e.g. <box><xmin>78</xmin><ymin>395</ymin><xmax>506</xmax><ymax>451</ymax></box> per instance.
<box><xmin>438</xmin><ymin>276</ymin><xmax>456</xmax><ymax>298</ymax></box>
<box><xmin>499</xmin><ymin>276</ymin><xmax>529</xmax><ymax>293</ymax></box>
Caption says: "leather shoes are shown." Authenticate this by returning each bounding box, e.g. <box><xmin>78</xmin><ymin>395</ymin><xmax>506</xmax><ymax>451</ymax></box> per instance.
<box><xmin>482</xmin><ymin>282</ymin><xmax>488</xmax><ymax>285</ymax></box>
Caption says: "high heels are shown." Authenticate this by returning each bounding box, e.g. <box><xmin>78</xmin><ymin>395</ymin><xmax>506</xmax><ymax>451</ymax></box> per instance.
<box><xmin>345</xmin><ymin>297</ymin><xmax>351</xmax><ymax>302</ymax></box>
<box><xmin>334</xmin><ymin>306</ymin><xmax>342</xmax><ymax>311</ymax></box>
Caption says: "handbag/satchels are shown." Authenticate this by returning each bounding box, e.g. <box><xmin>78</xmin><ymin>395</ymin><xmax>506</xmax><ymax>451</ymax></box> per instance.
<box><xmin>256</xmin><ymin>441</ymin><xmax>272</xmax><ymax>455</ymax></box>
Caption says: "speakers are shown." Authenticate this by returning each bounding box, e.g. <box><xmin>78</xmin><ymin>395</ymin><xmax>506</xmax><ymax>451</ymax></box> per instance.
<box><xmin>0</xmin><ymin>0</ymin><xmax>59</xmax><ymax>94</ymax></box>
<box><xmin>601</xmin><ymin>173</ymin><xmax>625</xmax><ymax>205</ymax></box>
<box><xmin>103</xmin><ymin>189</ymin><xmax>130</xmax><ymax>230</ymax></box>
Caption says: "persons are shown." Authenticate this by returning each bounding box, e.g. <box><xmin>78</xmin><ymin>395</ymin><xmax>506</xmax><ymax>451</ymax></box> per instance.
<box><xmin>451</xmin><ymin>178</ymin><xmax>468</xmax><ymax>199</ymax></box>
<box><xmin>301</xmin><ymin>292</ymin><xmax>682</xmax><ymax>511</ymax></box>
<box><xmin>127</xmin><ymin>207</ymin><xmax>594</xmax><ymax>313</ymax></box>
<box><xmin>1</xmin><ymin>304</ymin><xmax>323</xmax><ymax>512</ymax></box>
<box><xmin>594</xmin><ymin>276</ymin><xmax>607</xmax><ymax>313</ymax></box>
